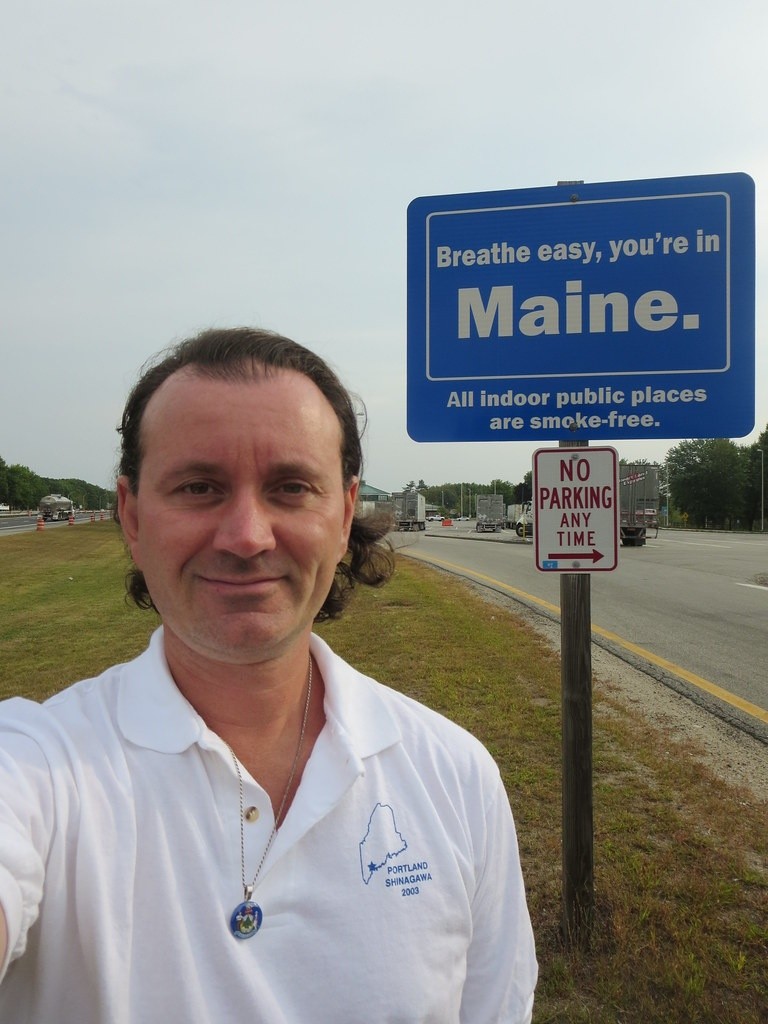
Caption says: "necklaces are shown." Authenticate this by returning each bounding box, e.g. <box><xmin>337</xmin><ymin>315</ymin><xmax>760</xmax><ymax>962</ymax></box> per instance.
<box><xmin>184</xmin><ymin>653</ymin><xmax>313</xmax><ymax>941</ymax></box>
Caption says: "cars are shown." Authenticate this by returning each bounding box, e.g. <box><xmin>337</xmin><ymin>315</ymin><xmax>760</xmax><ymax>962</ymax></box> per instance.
<box><xmin>457</xmin><ymin>516</ymin><xmax>470</xmax><ymax>521</ymax></box>
<box><xmin>0</xmin><ymin>503</ymin><xmax>9</xmax><ymax>511</ymax></box>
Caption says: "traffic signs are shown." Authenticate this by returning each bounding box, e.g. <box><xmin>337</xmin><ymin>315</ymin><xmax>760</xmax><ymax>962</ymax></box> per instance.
<box><xmin>407</xmin><ymin>171</ymin><xmax>757</xmax><ymax>570</ymax></box>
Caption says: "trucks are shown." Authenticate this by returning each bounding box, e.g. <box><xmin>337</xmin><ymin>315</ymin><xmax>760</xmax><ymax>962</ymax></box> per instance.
<box><xmin>392</xmin><ymin>492</ymin><xmax>426</xmax><ymax>532</ymax></box>
<box><xmin>37</xmin><ymin>494</ymin><xmax>76</xmax><ymax>521</ymax></box>
<box><xmin>474</xmin><ymin>494</ymin><xmax>503</xmax><ymax>534</ymax></box>
<box><xmin>619</xmin><ymin>463</ymin><xmax>660</xmax><ymax>547</ymax></box>
<box><xmin>506</xmin><ymin>504</ymin><xmax>523</xmax><ymax>529</ymax></box>
<box><xmin>516</xmin><ymin>498</ymin><xmax>533</xmax><ymax>537</ymax></box>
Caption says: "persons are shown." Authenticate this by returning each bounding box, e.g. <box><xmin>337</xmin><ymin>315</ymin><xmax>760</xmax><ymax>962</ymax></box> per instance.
<box><xmin>0</xmin><ymin>329</ymin><xmax>542</xmax><ymax>1024</ymax></box>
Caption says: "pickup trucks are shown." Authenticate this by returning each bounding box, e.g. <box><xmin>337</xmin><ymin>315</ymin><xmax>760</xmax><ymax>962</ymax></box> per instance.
<box><xmin>426</xmin><ymin>515</ymin><xmax>445</xmax><ymax>522</ymax></box>
